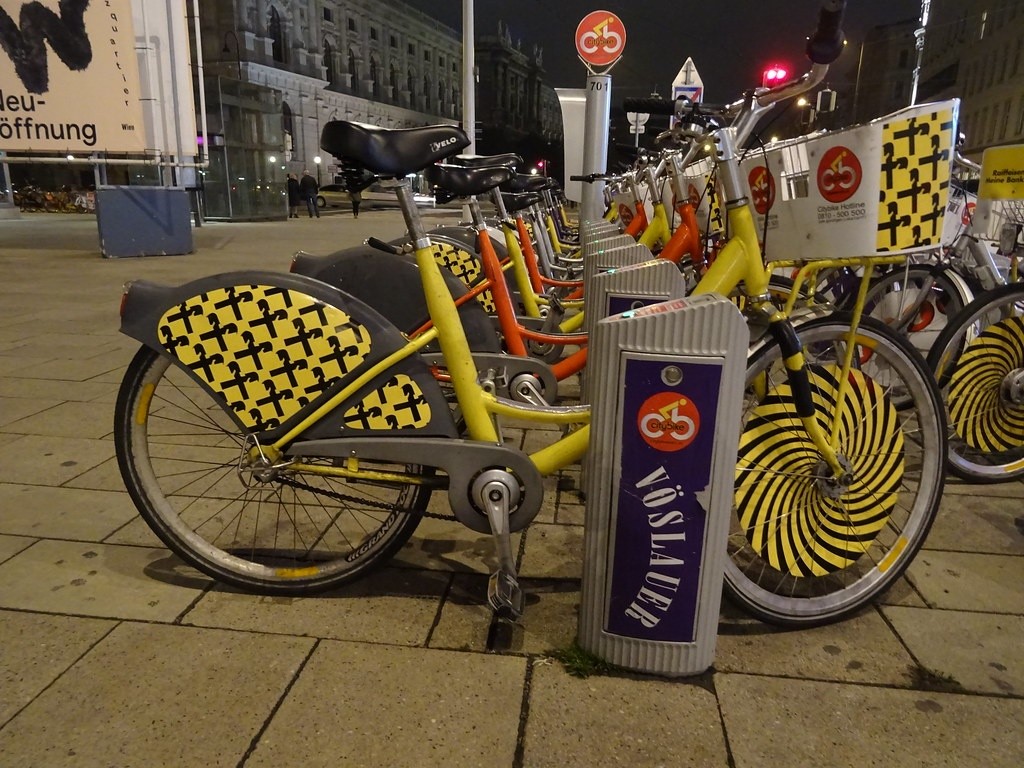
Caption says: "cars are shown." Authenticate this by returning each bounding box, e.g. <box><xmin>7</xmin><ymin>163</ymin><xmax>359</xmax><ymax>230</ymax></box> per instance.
<box><xmin>359</xmin><ymin>182</ymin><xmax>435</xmax><ymax>210</ymax></box>
<box><xmin>315</xmin><ymin>184</ymin><xmax>357</xmax><ymax>208</ymax></box>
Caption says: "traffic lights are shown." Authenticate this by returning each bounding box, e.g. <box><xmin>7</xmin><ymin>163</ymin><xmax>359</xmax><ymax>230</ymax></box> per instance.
<box><xmin>537</xmin><ymin>159</ymin><xmax>543</xmax><ymax>168</ymax></box>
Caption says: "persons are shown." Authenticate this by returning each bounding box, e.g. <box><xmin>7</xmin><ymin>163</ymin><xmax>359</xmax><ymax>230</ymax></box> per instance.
<box><xmin>300</xmin><ymin>169</ymin><xmax>320</xmax><ymax>218</ymax></box>
<box><xmin>349</xmin><ymin>184</ymin><xmax>362</xmax><ymax>219</ymax></box>
<box><xmin>288</xmin><ymin>173</ymin><xmax>301</xmax><ymax>218</ymax></box>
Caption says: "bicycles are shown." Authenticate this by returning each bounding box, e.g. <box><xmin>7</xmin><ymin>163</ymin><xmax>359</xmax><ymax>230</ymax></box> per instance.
<box><xmin>3</xmin><ymin>185</ymin><xmax>95</xmax><ymax>214</ymax></box>
<box><xmin>111</xmin><ymin>0</ymin><xmax>1024</xmax><ymax>627</ymax></box>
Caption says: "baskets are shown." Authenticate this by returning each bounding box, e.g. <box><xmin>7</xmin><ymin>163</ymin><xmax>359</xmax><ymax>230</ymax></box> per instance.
<box><xmin>971</xmin><ymin>145</ymin><xmax>1024</xmax><ymax>245</ymax></box>
<box><xmin>744</xmin><ymin>98</ymin><xmax>962</xmax><ymax>259</ymax></box>
<box><xmin>683</xmin><ymin>149</ymin><xmax>750</xmax><ymax>238</ymax></box>
<box><xmin>941</xmin><ymin>183</ymin><xmax>977</xmax><ymax>250</ymax></box>
